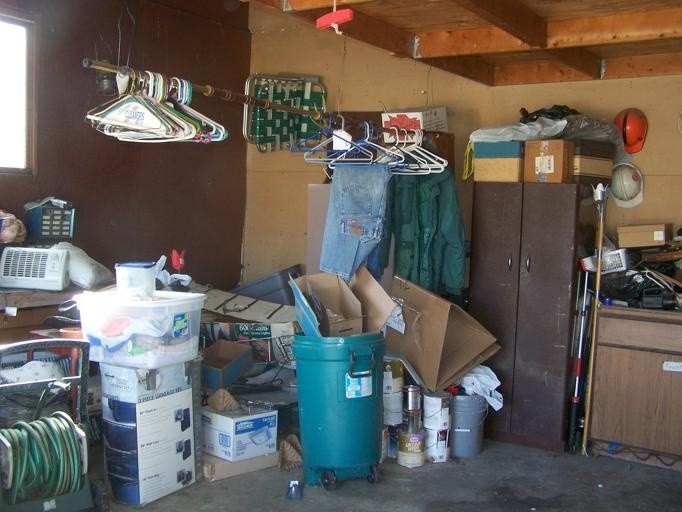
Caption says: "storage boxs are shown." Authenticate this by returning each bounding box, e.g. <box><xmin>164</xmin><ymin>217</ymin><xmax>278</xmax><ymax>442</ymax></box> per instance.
<box><xmin>287</xmin><ymin>263</ymin><xmax>394</xmax><ymax>341</ymax></box>
<box><xmin>618</xmin><ymin>219</ymin><xmax>671</xmax><ymax>250</ymax></box>
<box><xmin>473</xmin><ymin>133</ymin><xmax>614</xmax><ymax>188</ymax></box>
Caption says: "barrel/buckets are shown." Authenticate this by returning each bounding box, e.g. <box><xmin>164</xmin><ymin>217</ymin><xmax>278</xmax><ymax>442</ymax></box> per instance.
<box><xmin>423</xmin><ymin>429</ymin><xmax>451</xmax><ymax>463</ymax></box>
<box><xmin>422</xmin><ymin>392</ymin><xmax>451</xmax><ymax>429</ymax></box>
<box><xmin>397</xmin><ymin>428</ymin><xmax>428</xmax><ymax>468</ymax></box>
<box><xmin>383</xmin><ymin>358</ymin><xmax>405</xmax><ymax>426</ymax></box>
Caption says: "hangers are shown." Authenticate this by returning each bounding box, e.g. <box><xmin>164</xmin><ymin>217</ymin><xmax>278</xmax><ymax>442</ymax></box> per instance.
<box><xmin>85</xmin><ymin>59</ymin><xmax>227</xmax><ymax>144</ymax></box>
<box><xmin>290</xmin><ymin>113</ymin><xmax>448</xmax><ymax>177</ymax></box>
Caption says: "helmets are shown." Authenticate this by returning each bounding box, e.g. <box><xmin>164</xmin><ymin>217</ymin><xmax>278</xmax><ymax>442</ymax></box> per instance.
<box><xmin>610</xmin><ymin>106</ymin><xmax>649</xmax><ymax>155</ymax></box>
<box><xmin>607</xmin><ymin>161</ymin><xmax>646</xmax><ymax>210</ymax></box>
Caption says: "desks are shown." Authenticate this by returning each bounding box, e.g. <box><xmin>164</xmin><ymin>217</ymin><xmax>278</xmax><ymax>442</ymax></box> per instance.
<box><xmin>2</xmin><ymin>288</ymin><xmax>101</xmax><ymax>329</ymax></box>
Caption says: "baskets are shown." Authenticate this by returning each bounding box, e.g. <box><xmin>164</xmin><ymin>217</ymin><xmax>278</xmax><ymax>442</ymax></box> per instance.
<box><xmin>593</xmin><ymin>248</ymin><xmax>631</xmax><ymax>276</ymax></box>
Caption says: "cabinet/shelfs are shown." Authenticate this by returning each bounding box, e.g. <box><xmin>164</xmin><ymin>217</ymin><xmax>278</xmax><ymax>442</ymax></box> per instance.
<box><xmin>467</xmin><ymin>182</ymin><xmax>593</xmax><ymax>459</ymax></box>
<box><xmin>585</xmin><ymin>302</ymin><xmax>682</xmax><ymax>469</ymax></box>
